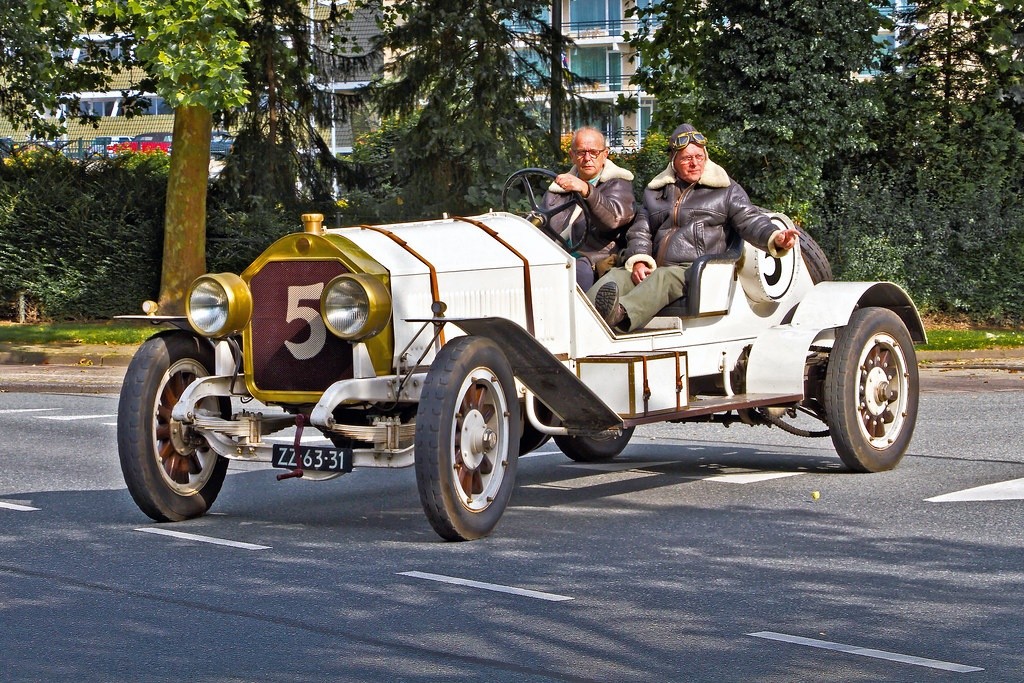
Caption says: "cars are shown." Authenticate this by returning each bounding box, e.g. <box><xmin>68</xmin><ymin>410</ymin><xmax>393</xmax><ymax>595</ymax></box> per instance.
<box><xmin>0</xmin><ymin>138</ymin><xmax>25</xmax><ymax>159</ymax></box>
<box><xmin>209</xmin><ymin>130</ymin><xmax>234</xmax><ymax>161</ymax></box>
<box><xmin>84</xmin><ymin>135</ymin><xmax>136</xmax><ymax>158</ymax></box>
<box><xmin>116</xmin><ymin>164</ymin><xmax>929</xmax><ymax>543</ymax></box>
<box><xmin>108</xmin><ymin>132</ymin><xmax>173</xmax><ymax>157</ymax></box>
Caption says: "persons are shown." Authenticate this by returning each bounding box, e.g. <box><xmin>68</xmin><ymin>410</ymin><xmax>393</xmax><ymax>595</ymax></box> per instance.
<box><xmin>586</xmin><ymin>123</ymin><xmax>801</xmax><ymax>334</ymax></box>
<box><xmin>538</xmin><ymin>126</ymin><xmax>637</xmax><ymax>293</ymax></box>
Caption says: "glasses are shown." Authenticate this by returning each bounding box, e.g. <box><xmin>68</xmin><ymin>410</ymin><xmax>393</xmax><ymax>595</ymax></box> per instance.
<box><xmin>673</xmin><ymin>132</ymin><xmax>707</xmax><ymax>150</ymax></box>
<box><xmin>571</xmin><ymin>148</ymin><xmax>606</xmax><ymax>159</ymax></box>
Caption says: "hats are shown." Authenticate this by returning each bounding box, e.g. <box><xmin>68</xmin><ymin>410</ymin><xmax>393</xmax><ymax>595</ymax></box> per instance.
<box><xmin>669</xmin><ymin>124</ymin><xmax>708</xmax><ymax>173</ymax></box>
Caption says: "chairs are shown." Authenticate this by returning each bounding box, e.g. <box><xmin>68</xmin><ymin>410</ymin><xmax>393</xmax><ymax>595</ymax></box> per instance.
<box><xmin>656</xmin><ymin>240</ymin><xmax>741</xmax><ymax>318</ymax></box>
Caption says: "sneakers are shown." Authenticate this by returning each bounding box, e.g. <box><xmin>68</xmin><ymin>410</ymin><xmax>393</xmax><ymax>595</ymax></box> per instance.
<box><xmin>595</xmin><ymin>281</ymin><xmax>628</xmax><ymax>327</ymax></box>
